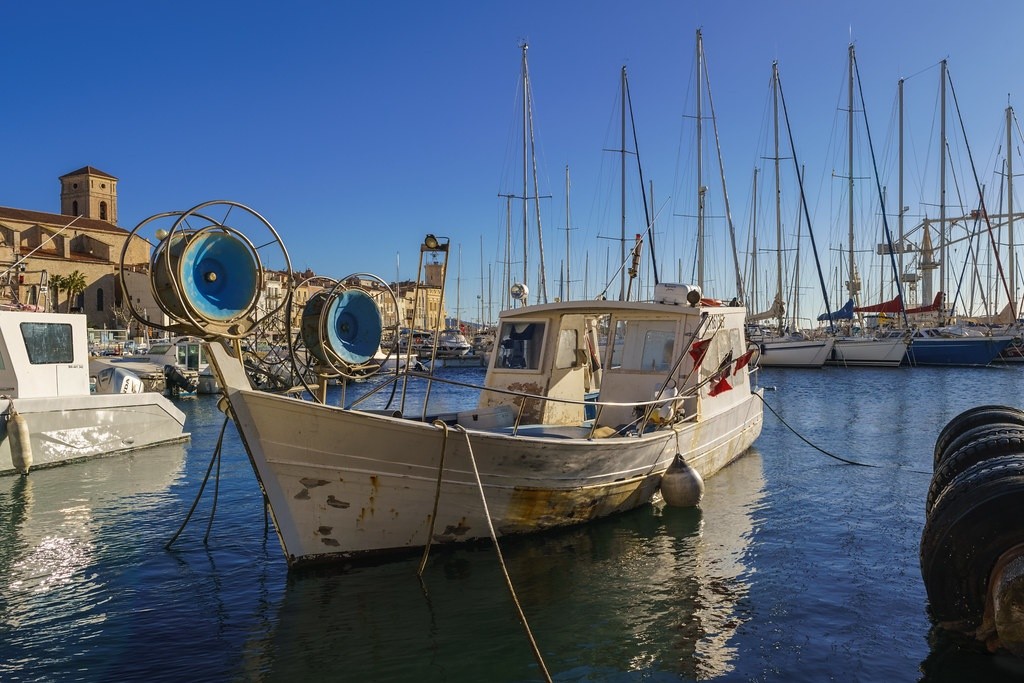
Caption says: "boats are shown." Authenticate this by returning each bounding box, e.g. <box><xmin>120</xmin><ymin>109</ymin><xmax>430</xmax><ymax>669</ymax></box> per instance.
<box><xmin>0</xmin><ymin>310</ymin><xmax>191</xmax><ymax>476</ymax></box>
<box><xmin>126</xmin><ymin>200</ymin><xmax>764</xmax><ymax>566</ymax></box>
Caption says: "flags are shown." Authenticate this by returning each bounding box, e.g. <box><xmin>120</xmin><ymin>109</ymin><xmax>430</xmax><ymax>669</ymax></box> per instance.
<box><xmin>688</xmin><ymin>341</ymin><xmax>756</xmax><ymax>397</ymax></box>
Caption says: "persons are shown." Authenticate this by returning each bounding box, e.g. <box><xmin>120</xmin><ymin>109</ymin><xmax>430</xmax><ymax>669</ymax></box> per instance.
<box><xmin>664</xmin><ymin>339</ymin><xmax>674</xmax><ymax>363</ymax></box>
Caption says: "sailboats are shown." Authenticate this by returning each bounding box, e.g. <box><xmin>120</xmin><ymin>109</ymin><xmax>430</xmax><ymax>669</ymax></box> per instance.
<box><xmin>419</xmin><ymin>26</ymin><xmax>1023</xmax><ymax>371</ymax></box>
